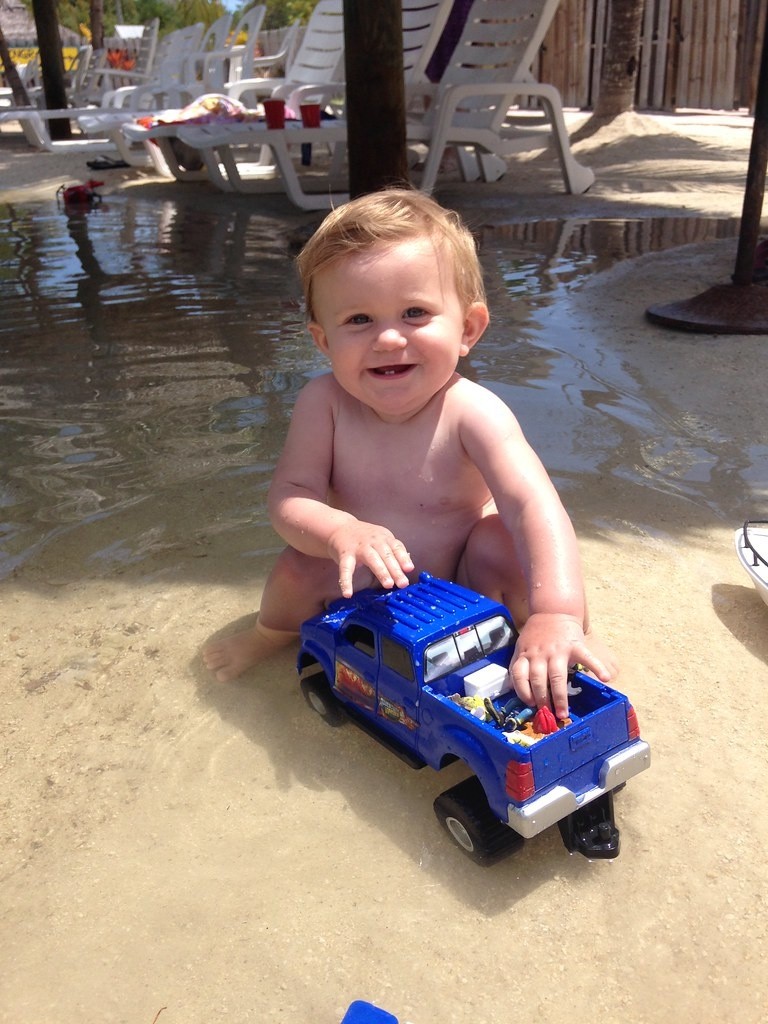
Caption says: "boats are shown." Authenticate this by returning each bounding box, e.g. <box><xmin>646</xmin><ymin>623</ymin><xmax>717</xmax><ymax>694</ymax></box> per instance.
<box><xmin>735</xmin><ymin>519</ymin><xmax>768</xmax><ymax>606</ymax></box>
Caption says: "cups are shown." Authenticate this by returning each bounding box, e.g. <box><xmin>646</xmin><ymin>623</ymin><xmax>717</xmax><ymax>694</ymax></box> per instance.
<box><xmin>263</xmin><ymin>100</ymin><xmax>285</xmax><ymax>129</ymax></box>
<box><xmin>299</xmin><ymin>104</ymin><xmax>321</xmax><ymax>128</ymax></box>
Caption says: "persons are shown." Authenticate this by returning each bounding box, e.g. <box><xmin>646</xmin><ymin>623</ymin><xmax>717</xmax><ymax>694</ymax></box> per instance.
<box><xmin>200</xmin><ymin>189</ymin><xmax>612</xmax><ymax>721</ymax></box>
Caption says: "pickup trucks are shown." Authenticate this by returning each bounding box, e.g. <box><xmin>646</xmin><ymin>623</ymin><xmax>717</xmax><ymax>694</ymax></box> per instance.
<box><xmin>297</xmin><ymin>571</ymin><xmax>652</xmax><ymax>862</ymax></box>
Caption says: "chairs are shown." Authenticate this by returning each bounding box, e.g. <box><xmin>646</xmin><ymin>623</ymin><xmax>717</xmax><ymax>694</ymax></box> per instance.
<box><xmin>177</xmin><ymin>0</ymin><xmax>595</xmax><ymax>212</ymax></box>
<box><xmin>253</xmin><ymin>17</ymin><xmax>300</xmax><ymax>78</ymax></box>
<box><xmin>2</xmin><ymin>59</ymin><xmax>39</xmax><ymax>105</ymax></box>
<box><xmin>120</xmin><ymin>0</ymin><xmax>456</xmax><ymax>195</ymax></box>
<box><xmin>29</xmin><ymin>48</ymin><xmax>91</xmax><ymax>112</ymax></box>
<box><xmin>78</xmin><ymin>0</ymin><xmax>346</xmax><ymax>178</ymax></box>
<box><xmin>4</xmin><ymin>23</ymin><xmax>203</xmax><ymax>153</ymax></box>
<box><xmin>89</xmin><ymin>16</ymin><xmax>160</xmax><ymax>100</ymax></box>
<box><xmin>180</xmin><ymin>12</ymin><xmax>230</xmax><ymax>85</ymax></box>
<box><xmin>193</xmin><ymin>4</ymin><xmax>267</xmax><ymax>87</ymax></box>
<box><xmin>103</xmin><ymin>29</ymin><xmax>179</xmax><ymax>108</ymax></box>
